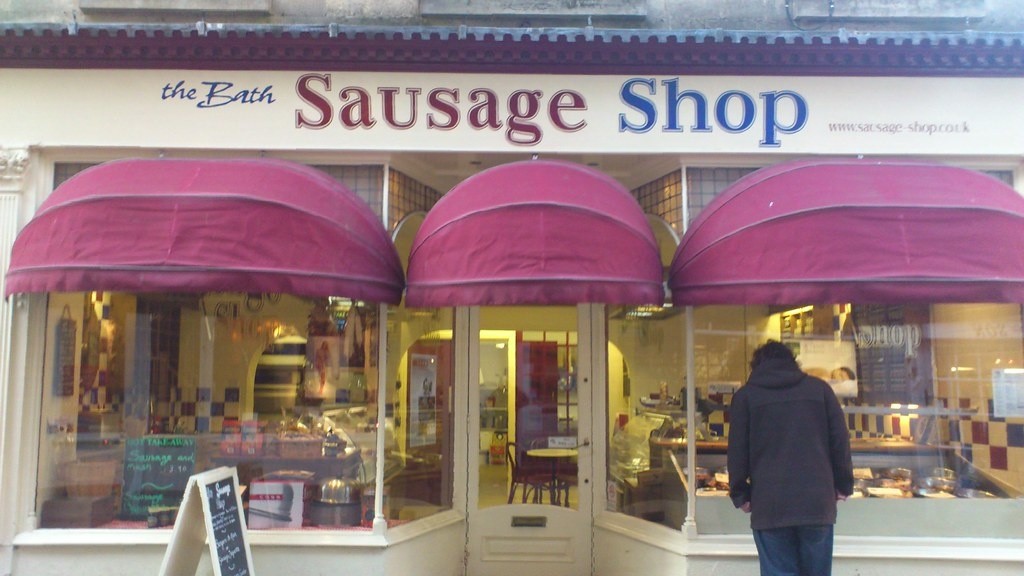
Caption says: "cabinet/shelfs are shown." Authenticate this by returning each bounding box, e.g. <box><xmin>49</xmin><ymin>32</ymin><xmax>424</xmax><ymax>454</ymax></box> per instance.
<box><xmin>780</xmin><ymin>303</ymin><xmax>834</xmax><ymax>336</ymax></box>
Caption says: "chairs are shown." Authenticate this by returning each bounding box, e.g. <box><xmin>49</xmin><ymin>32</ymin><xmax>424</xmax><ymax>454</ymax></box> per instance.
<box><xmin>506</xmin><ymin>438</ymin><xmax>577</xmax><ymax>508</ymax></box>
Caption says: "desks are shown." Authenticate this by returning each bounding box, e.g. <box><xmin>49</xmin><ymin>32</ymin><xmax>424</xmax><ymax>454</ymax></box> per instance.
<box><xmin>526</xmin><ymin>449</ymin><xmax>578</xmax><ymax>505</ymax></box>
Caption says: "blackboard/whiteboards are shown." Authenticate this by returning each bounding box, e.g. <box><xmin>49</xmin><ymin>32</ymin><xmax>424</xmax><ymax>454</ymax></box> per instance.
<box><xmin>117</xmin><ymin>434</ymin><xmax>198</xmax><ymax>522</ymax></box>
<box><xmin>156</xmin><ymin>466</ymin><xmax>255</xmax><ymax>576</ymax></box>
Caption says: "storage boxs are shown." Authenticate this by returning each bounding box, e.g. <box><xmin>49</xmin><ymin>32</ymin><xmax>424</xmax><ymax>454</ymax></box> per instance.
<box><xmin>241</xmin><ymin>420</ymin><xmax>267</xmax><ymax>457</ymax></box>
<box><xmin>219</xmin><ymin>420</ymin><xmax>242</xmax><ymax>456</ymax></box>
<box><xmin>41</xmin><ymin>496</ymin><xmax>115</xmax><ymax>529</ymax></box>
<box><xmin>248</xmin><ymin>469</ymin><xmax>317</xmax><ymax>530</ymax></box>
<box><xmin>277</xmin><ymin>437</ymin><xmax>325</xmax><ymax>458</ymax></box>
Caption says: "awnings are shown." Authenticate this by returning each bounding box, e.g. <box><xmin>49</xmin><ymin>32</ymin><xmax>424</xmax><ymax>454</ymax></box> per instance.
<box><xmin>1</xmin><ymin>157</ymin><xmax>406</xmax><ymax>308</ymax></box>
<box><xmin>405</xmin><ymin>161</ymin><xmax>665</xmax><ymax>308</ymax></box>
<box><xmin>667</xmin><ymin>157</ymin><xmax>1024</xmax><ymax>308</ymax></box>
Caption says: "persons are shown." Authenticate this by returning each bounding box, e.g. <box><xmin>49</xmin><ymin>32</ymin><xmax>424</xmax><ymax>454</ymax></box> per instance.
<box><xmin>727</xmin><ymin>339</ymin><xmax>855</xmax><ymax>576</ymax></box>
